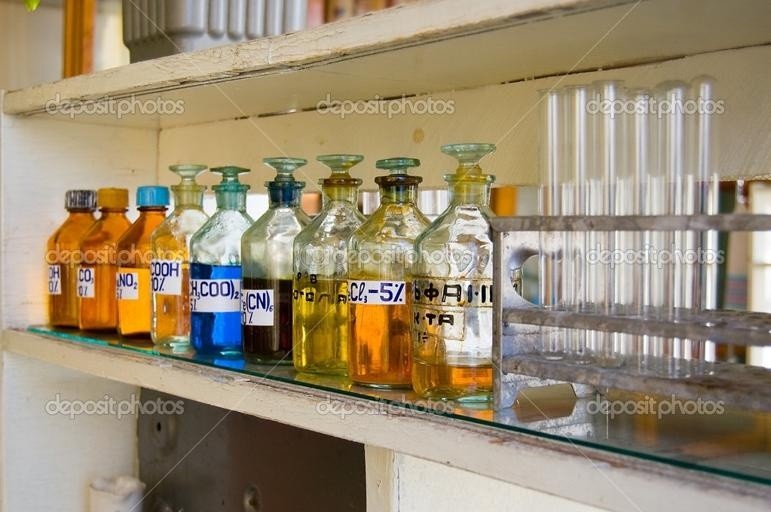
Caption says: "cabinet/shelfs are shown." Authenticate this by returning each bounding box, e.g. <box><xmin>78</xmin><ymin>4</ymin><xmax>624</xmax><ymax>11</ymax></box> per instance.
<box><xmin>0</xmin><ymin>1</ymin><xmax>771</xmax><ymax>511</ymax></box>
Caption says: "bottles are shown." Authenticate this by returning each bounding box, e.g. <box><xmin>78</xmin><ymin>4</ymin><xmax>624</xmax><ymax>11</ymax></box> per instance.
<box><xmin>531</xmin><ymin>73</ymin><xmax>721</xmax><ymax>375</ymax></box>
<box><xmin>155</xmin><ymin>151</ymin><xmax>437</xmax><ymax>393</ymax></box>
<box><xmin>46</xmin><ymin>185</ymin><xmax>188</xmax><ymax>344</ymax></box>
<box><xmin>414</xmin><ymin>141</ymin><xmax>505</xmax><ymax>398</ymax></box>
